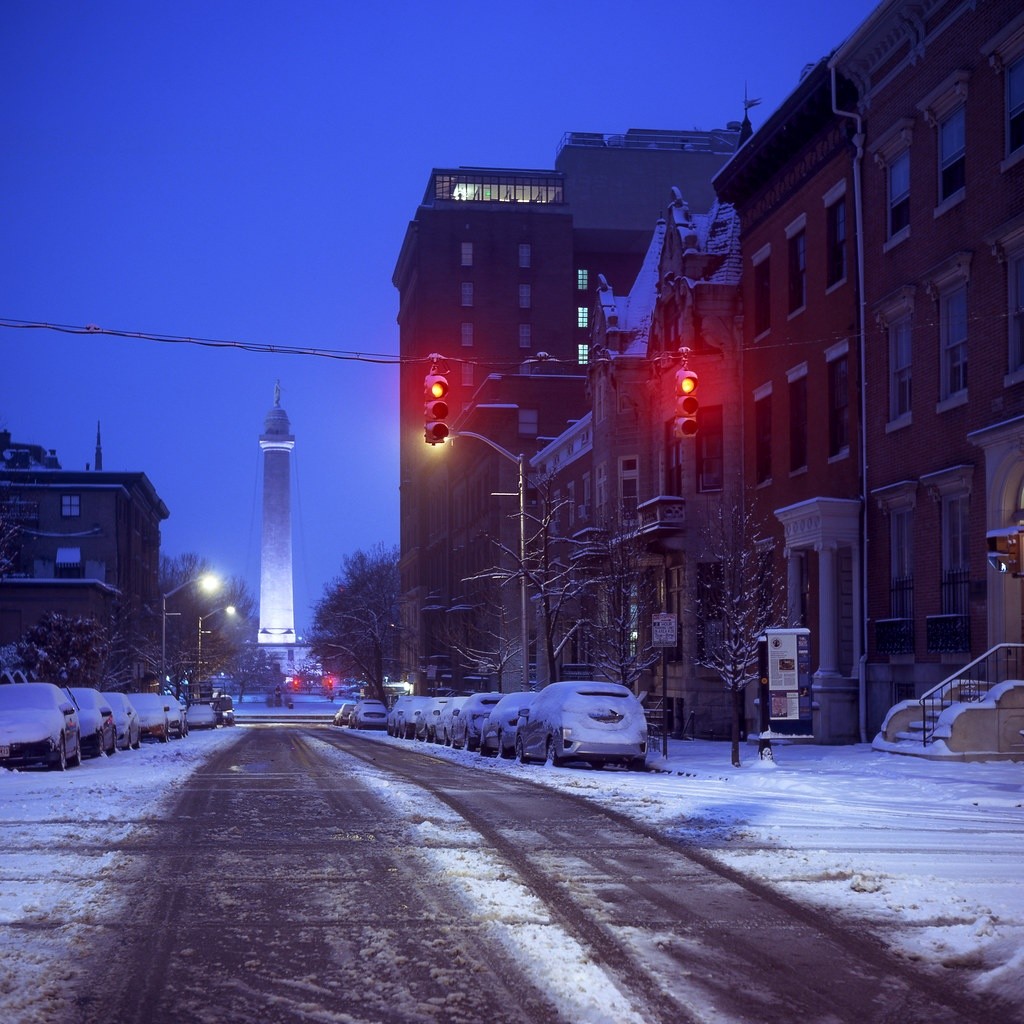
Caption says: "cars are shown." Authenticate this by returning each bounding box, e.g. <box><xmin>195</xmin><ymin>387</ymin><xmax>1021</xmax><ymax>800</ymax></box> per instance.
<box><xmin>513</xmin><ymin>679</ymin><xmax>649</xmax><ymax>770</ymax></box>
<box><xmin>482</xmin><ymin>690</ymin><xmax>538</xmax><ymax>757</ymax></box>
<box><xmin>125</xmin><ymin>693</ymin><xmax>170</xmax><ymax>744</ymax></box>
<box><xmin>385</xmin><ymin>695</ymin><xmax>418</xmax><ymax>736</ymax></box>
<box><xmin>98</xmin><ymin>692</ymin><xmax>139</xmax><ymax>749</ymax></box>
<box><xmin>397</xmin><ymin>697</ymin><xmax>429</xmax><ymax>738</ymax></box>
<box><xmin>58</xmin><ymin>686</ymin><xmax>115</xmax><ymax>755</ymax></box>
<box><xmin>452</xmin><ymin>693</ymin><xmax>508</xmax><ymax>752</ymax></box>
<box><xmin>334</xmin><ymin>702</ymin><xmax>388</xmax><ymax>730</ymax></box>
<box><xmin>414</xmin><ymin>699</ymin><xmax>451</xmax><ymax>741</ymax></box>
<box><xmin>160</xmin><ymin>694</ymin><xmax>190</xmax><ymax>739</ymax></box>
<box><xmin>432</xmin><ymin>697</ymin><xmax>470</xmax><ymax>748</ymax></box>
<box><xmin>0</xmin><ymin>681</ymin><xmax>83</xmax><ymax>770</ymax></box>
<box><xmin>184</xmin><ymin>704</ymin><xmax>217</xmax><ymax>730</ymax></box>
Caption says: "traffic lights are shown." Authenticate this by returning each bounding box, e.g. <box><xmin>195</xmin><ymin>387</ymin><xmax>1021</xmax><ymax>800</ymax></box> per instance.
<box><xmin>675</xmin><ymin>368</ymin><xmax>701</xmax><ymax>439</ymax></box>
<box><xmin>425</xmin><ymin>374</ymin><xmax>450</xmax><ymax>445</ymax></box>
<box><xmin>327</xmin><ymin>678</ymin><xmax>334</xmax><ymax>690</ymax></box>
<box><xmin>294</xmin><ymin>679</ymin><xmax>299</xmax><ymax>691</ymax></box>
<box><xmin>987</xmin><ymin>530</ymin><xmax>1012</xmax><ymax>573</ymax></box>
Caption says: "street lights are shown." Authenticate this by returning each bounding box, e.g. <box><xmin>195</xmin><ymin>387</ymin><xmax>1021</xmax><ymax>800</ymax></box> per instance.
<box><xmin>196</xmin><ymin>604</ymin><xmax>237</xmax><ymax>695</ymax></box>
<box><xmin>158</xmin><ymin>567</ymin><xmax>222</xmax><ymax>694</ymax></box>
